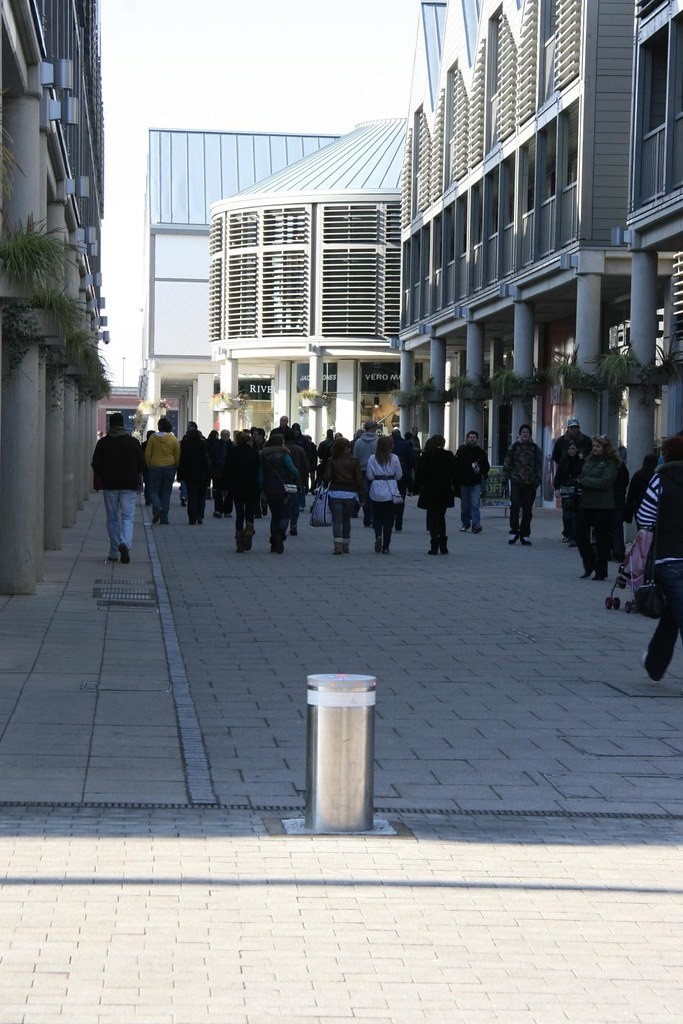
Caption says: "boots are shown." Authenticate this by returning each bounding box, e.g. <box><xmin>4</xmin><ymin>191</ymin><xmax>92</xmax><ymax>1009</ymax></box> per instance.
<box><xmin>332</xmin><ymin>538</ymin><xmax>351</xmax><ymax>555</ymax></box>
<box><xmin>427</xmin><ymin>536</ymin><xmax>450</xmax><ymax>555</ymax></box>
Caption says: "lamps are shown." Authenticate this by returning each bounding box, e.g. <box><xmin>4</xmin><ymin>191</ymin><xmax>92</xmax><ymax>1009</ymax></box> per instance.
<box><xmin>374</xmin><ymin>396</ymin><xmax>380</xmax><ymax>408</ymax></box>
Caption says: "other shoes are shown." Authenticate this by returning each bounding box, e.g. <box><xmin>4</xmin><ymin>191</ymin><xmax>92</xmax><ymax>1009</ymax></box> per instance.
<box><xmin>151</xmin><ymin>508</ymin><xmax>169</xmax><ymax>525</ymax></box>
<box><xmin>519</xmin><ymin>534</ymin><xmax>532</xmax><ymax>546</ymax></box>
<box><xmin>374</xmin><ymin>541</ymin><xmax>390</xmax><ymax>555</ymax></box>
<box><xmin>188</xmin><ymin>518</ymin><xmax>204</xmax><ymax>526</ymax></box>
<box><xmin>580</xmin><ymin>572</ymin><xmax>605</xmax><ymax>581</ymax></box>
<box><xmin>213</xmin><ymin>511</ymin><xmax>233</xmax><ymax>518</ymax></box>
<box><xmin>289</xmin><ymin>526</ymin><xmax>298</xmax><ymax>536</ymax></box>
<box><xmin>460</xmin><ymin>521</ymin><xmax>483</xmax><ymax>534</ymax></box>
<box><xmin>270</xmin><ymin>535</ymin><xmax>286</xmax><ymax>553</ymax></box>
<box><xmin>509</xmin><ymin>534</ymin><xmax>519</xmax><ymax>544</ymax></box>
<box><xmin>641</xmin><ymin>651</ymin><xmax>659</xmax><ymax>682</ymax></box>
<box><xmin>253</xmin><ymin>502</ymin><xmax>268</xmax><ymax>520</ymax></box>
<box><xmin>234</xmin><ymin>527</ymin><xmax>256</xmax><ymax>554</ymax></box>
<box><xmin>108</xmin><ymin>542</ymin><xmax>130</xmax><ymax>564</ymax></box>
<box><xmin>180</xmin><ymin>496</ymin><xmax>186</xmax><ymax>507</ymax></box>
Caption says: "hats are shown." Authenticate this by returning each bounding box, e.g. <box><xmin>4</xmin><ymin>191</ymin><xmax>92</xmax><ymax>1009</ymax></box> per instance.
<box><xmin>567</xmin><ymin>418</ymin><xmax>579</xmax><ymax>428</ymax></box>
<box><xmin>364</xmin><ymin>421</ymin><xmax>377</xmax><ymax>432</ymax></box>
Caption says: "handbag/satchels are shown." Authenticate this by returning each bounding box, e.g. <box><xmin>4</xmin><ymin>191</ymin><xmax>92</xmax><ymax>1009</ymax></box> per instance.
<box><xmin>309</xmin><ymin>480</ymin><xmax>333</xmax><ymax>527</ymax></box>
<box><xmin>392</xmin><ymin>494</ymin><xmax>403</xmax><ymax>504</ymax></box>
<box><xmin>284</xmin><ymin>484</ymin><xmax>298</xmax><ymax>493</ymax></box>
<box><xmin>634</xmin><ymin>582</ymin><xmax>666</xmax><ymax>619</ymax></box>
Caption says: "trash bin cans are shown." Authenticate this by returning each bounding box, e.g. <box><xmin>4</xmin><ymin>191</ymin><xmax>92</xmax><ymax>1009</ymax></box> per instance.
<box><xmin>306</xmin><ymin>673</ymin><xmax>378</xmax><ymax>834</ymax></box>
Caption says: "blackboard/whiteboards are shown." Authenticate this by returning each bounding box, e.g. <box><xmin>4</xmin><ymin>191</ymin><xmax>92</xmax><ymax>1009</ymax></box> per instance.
<box><xmin>480</xmin><ymin>466</ymin><xmax>511</xmax><ymax>508</ymax></box>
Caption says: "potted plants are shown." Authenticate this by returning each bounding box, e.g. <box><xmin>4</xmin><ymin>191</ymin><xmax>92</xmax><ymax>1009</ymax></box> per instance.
<box><xmin>300</xmin><ymin>390</ymin><xmax>323</xmax><ymax>407</ymax></box>
<box><xmin>136</xmin><ymin>398</ymin><xmax>170</xmax><ymax>416</ymax></box>
<box><xmin>388</xmin><ymin>333</ymin><xmax>683</xmax><ymax>409</ymax></box>
<box><xmin>211</xmin><ymin>393</ymin><xmax>250</xmax><ymax>412</ymax></box>
<box><xmin>0</xmin><ymin>215</ymin><xmax>112</xmax><ymax>402</ymax></box>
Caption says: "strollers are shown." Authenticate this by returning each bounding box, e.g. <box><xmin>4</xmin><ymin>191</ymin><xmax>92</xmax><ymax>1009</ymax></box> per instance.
<box><xmin>605</xmin><ymin>529</ymin><xmax>653</xmax><ymax>614</ymax></box>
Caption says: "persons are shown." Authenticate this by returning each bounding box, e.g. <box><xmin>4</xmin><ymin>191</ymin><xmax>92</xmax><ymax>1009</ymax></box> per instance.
<box><xmin>91</xmin><ymin>413</ymin><xmax>144</xmax><ymax>564</ymax></box>
<box><xmin>364</xmin><ymin>436</ymin><xmax>404</xmax><ymax>554</ymax></box>
<box><xmin>323</xmin><ymin>439</ymin><xmax>367</xmax><ymax>555</ymax></box>
<box><xmin>259</xmin><ymin>433</ymin><xmax>300</xmax><ymax>554</ymax></box>
<box><xmin>625</xmin><ymin>431</ymin><xmax>683</xmax><ymax>684</ymax></box>
<box><xmin>141</xmin><ymin>417</ymin><xmax>424</xmax><ymax>533</ymax></box>
<box><xmin>413</xmin><ymin>431</ymin><xmax>490</xmax><ymax>556</ymax></box>
<box><xmin>499</xmin><ymin>424</ymin><xmax>544</xmax><ymax>545</ymax></box>
<box><xmin>552</xmin><ymin>419</ymin><xmax>629</xmax><ymax>580</ymax></box>
<box><xmin>221</xmin><ymin>431</ymin><xmax>261</xmax><ymax>554</ymax></box>
